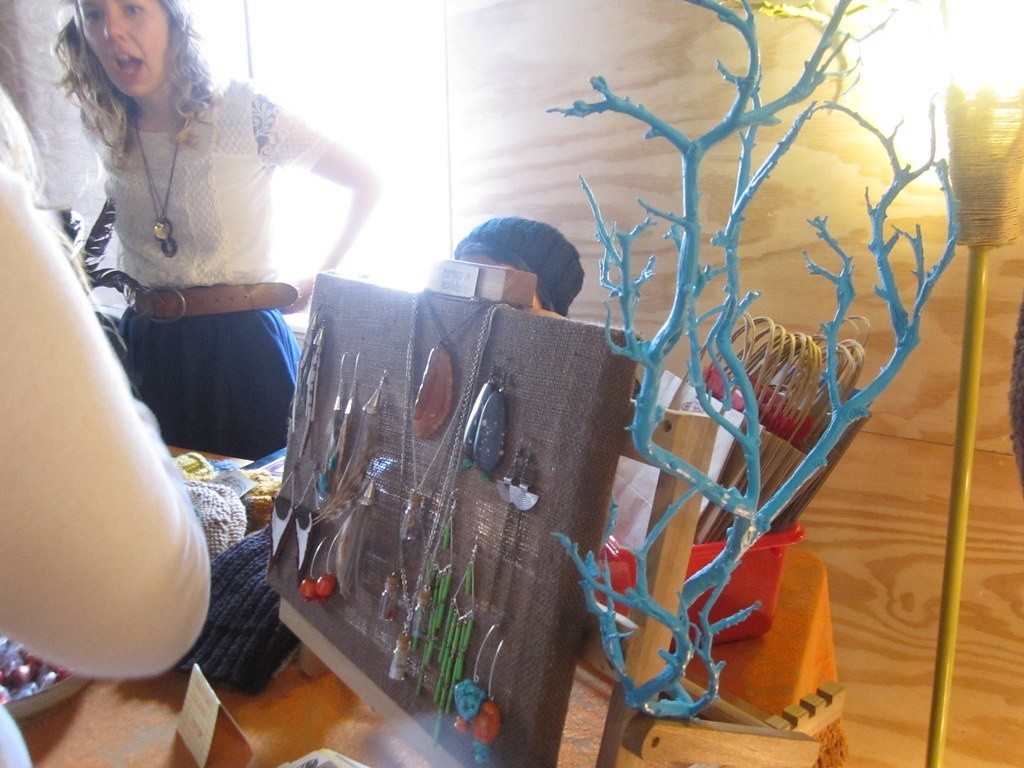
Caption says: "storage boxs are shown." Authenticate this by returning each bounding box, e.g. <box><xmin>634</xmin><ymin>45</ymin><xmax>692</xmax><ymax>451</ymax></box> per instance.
<box><xmin>668</xmin><ymin>521</ymin><xmax>806</xmax><ymax>656</ymax></box>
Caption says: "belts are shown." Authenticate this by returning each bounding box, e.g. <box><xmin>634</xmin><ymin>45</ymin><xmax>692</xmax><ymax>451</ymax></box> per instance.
<box><xmin>121</xmin><ymin>279</ymin><xmax>298</xmax><ymax>324</ymax></box>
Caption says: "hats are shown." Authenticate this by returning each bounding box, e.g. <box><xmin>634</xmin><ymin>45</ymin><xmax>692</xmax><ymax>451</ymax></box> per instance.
<box><xmin>173</xmin><ymin>524</ymin><xmax>299</xmax><ymax>693</ymax></box>
<box><xmin>176</xmin><ymin>450</ymin><xmax>213</xmax><ymax>482</ymax></box>
<box><xmin>243</xmin><ymin>467</ymin><xmax>284</xmax><ymax>533</ymax></box>
<box><xmin>189</xmin><ymin>483</ymin><xmax>247</xmax><ymax>559</ymax></box>
<box><xmin>455</xmin><ymin>215</ymin><xmax>585</xmax><ymax>317</ymax></box>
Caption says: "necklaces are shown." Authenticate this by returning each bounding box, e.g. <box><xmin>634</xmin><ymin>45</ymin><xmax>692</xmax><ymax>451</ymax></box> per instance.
<box><xmin>135</xmin><ymin>109</ymin><xmax>186</xmax><ymax>257</ymax></box>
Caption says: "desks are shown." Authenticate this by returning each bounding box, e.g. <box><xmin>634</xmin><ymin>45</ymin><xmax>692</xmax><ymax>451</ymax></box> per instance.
<box><xmin>22</xmin><ymin>439</ymin><xmax>691</xmax><ymax>768</ymax></box>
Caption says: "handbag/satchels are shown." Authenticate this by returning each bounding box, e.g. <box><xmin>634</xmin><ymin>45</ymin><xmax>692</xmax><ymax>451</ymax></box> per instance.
<box><xmin>696</xmin><ymin>310</ymin><xmax>871</xmax><ymax>547</ymax></box>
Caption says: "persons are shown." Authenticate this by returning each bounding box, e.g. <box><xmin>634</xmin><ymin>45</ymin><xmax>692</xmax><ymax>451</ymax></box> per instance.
<box><xmin>1</xmin><ymin>0</ymin><xmax>212</xmax><ymax>768</ymax></box>
<box><xmin>50</xmin><ymin>0</ymin><xmax>385</xmax><ymax>460</ymax></box>
<box><xmin>453</xmin><ymin>217</ymin><xmax>585</xmax><ymax>317</ymax></box>
